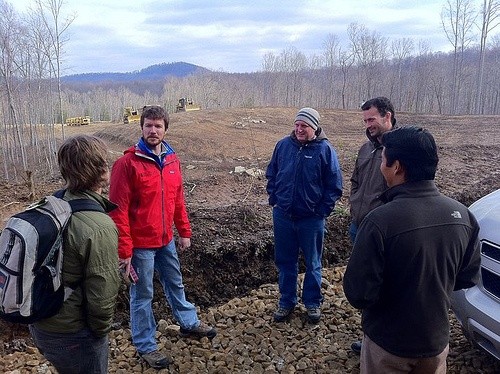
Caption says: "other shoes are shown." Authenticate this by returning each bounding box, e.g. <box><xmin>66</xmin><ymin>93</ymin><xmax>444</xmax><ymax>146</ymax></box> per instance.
<box><xmin>352</xmin><ymin>340</ymin><xmax>361</xmax><ymax>354</ymax></box>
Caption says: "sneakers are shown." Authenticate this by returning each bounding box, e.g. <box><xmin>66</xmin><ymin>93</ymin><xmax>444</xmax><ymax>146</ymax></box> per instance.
<box><xmin>139</xmin><ymin>350</ymin><xmax>170</xmax><ymax>369</ymax></box>
<box><xmin>274</xmin><ymin>307</ymin><xmax>295</xmax><ymax>321</ymax></box>
<box><xmin>179</xmin><ymin>319</ymin><xmax>217</xmax><ymax>338</ymax></box>
<box><xmin>307</xmin><ymin>306</ymin><xmax>321</xmax><ymax>324</ymax></box>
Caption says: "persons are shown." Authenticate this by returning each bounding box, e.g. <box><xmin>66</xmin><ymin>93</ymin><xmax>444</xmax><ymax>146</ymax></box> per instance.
<box><xmin>348</xmin><ymin>97</ymin><xmax>397</xmax><ymax>354</ymax></box>
<box><xmin>266</xmin><ymin>107</ymin><xmax>343</xmax><ymax>324</ymax></box>
<box><xmin>29</xmin><ymin>134</ymin><xmax>120</xmax><ymax>374</ymax></box>
<box><xmin>109</xmin><ymin>106</ymin><xmax>216</xmax><ymax>369</ymax></box>
<box><xmin>342</xmin><ymin>125</ymin><xmax>481</xmax><ymax>374</ymax></box>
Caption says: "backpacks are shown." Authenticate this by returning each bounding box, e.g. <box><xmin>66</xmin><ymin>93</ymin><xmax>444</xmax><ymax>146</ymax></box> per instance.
<box><xmin>0</xmin><ymin>189</ymin><xmax>107</xmax><ymax>323</ymax></box>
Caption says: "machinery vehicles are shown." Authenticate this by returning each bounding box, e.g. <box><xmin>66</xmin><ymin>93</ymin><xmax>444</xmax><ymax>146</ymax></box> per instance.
<box><xmin>122</xmin><ymin>106</ymin><xmax>141</xmax><ymax>125</ymax></box>
<box><xmin>175</xmin><ymin>97</ymin><xmax>200</xmax><ymax>114</ymax></box>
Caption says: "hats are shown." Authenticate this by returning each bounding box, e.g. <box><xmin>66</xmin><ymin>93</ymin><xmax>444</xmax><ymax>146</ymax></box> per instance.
<box><xmin>294</xmin><ymin>108</ymin><xmax>320</xmax><ymax>130</ymax></box>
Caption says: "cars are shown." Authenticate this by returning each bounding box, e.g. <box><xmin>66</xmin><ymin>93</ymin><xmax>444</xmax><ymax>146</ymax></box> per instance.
<box><xmin>447</xmin><ymin>188</ymin><xmax>500</xmax><ymax>362</ymax></box>
